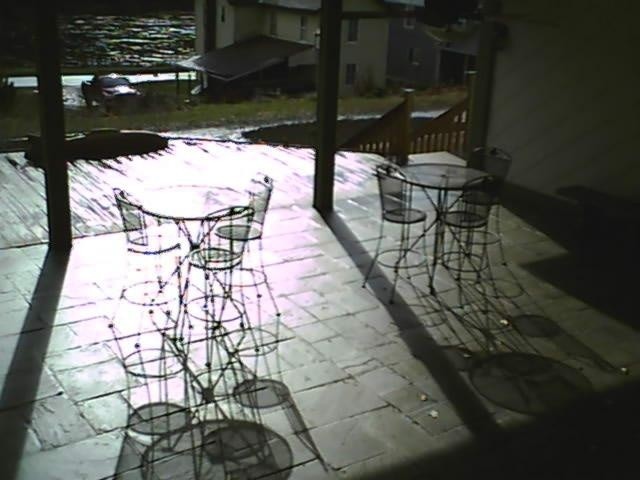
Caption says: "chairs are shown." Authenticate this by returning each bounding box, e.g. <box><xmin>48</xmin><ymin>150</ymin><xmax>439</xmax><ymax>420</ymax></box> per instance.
<box><xmin>360</xmin><ymin>145</ymin><xmax>518</xmax><ymax>307</ymax></box>
<box><xmin>110</xmin><ymin>170</ymin><xmax>281</xmax><ymax>332</ymax></box>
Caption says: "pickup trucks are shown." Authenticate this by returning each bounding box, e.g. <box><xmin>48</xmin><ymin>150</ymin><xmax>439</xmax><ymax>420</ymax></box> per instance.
<box><xmin>82</xmin><ymin>74</ymin><xmax>145</xmax><ymax>113</ymax></box>
